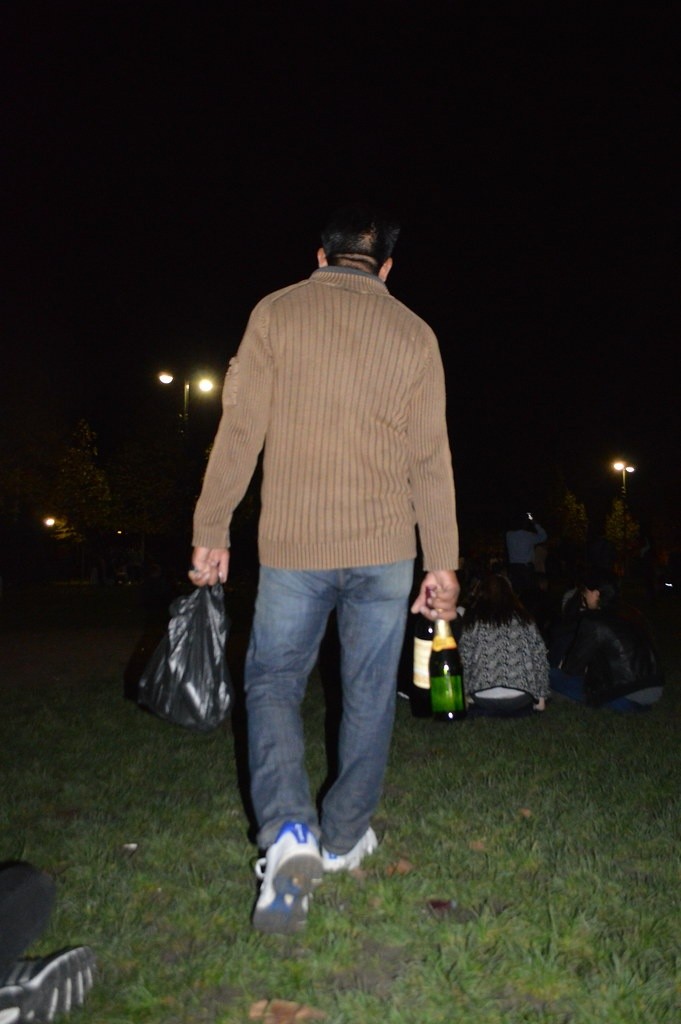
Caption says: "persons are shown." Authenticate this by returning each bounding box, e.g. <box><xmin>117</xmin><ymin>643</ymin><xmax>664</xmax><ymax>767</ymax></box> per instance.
<box><xmin>459</xmin><ymin>513</ymin><xmax>667</xmax><ymax>719</ymax></box>
<box><xmin>0</xmin><ymin>861</ymin><xmax>103</xmax><ymax>1024</ymax></box>
<box><xmin>189</xmin><ymin>204</ymin><xmax>463</xmax><ymax>929</ymax></box>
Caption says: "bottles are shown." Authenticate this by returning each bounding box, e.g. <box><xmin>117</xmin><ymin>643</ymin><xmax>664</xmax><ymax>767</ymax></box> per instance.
<box><xmin>414</xmin><ymin>587</ymin><xmax>465</xmax><ymax>725</ymax></box>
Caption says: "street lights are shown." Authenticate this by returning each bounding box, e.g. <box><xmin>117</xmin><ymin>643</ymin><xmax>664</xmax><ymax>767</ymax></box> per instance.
<box><xmin>158</xmin><ymin>371</ymin><xmax>219</xmax><ymax>440</ymax></box>
<box><xmin>611</xmin><ymin>460</ymin><xmax>635</xmax><ymax>578</ymax></box>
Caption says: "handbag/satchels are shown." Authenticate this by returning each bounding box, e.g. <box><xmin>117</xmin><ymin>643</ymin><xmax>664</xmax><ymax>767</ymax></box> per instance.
<box><xmin>135</xmin><ymin>572</ymin><xmax>236</xmax><ymax>732</ymax></box>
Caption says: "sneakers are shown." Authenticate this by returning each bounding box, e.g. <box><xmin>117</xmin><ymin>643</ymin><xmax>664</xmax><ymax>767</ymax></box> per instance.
<box><xmin>251</xmin><ymin>820</ymin><xmax>324</xmax><ymax>936</ymax></box>
<box><xmin>0</xmin><ymin>945</ymin><xmax>97</xmax><ymax>1024</ymax></box>
<box><xmin>322</xmin><ymin>826</ymin><xmax>377</xmax><ymax>874</ymax></box>
